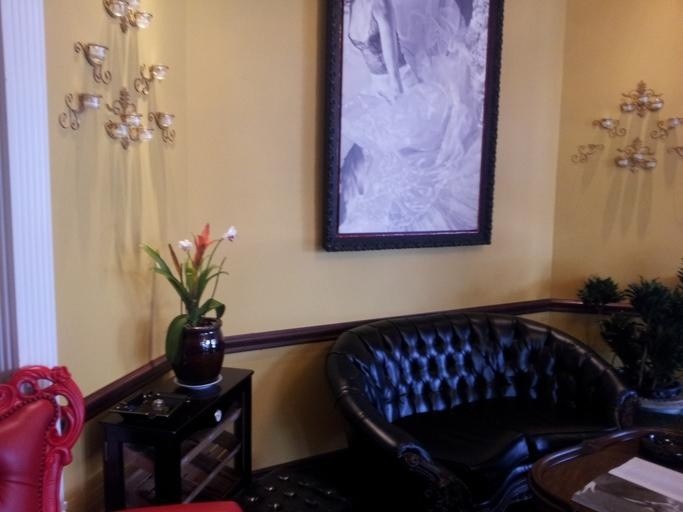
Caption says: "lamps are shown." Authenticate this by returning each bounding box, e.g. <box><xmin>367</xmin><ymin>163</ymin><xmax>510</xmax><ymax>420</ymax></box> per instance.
<box><xmin>58</xmin><ymin>0</ymin><xmax>179</xmax><ymax>151</ymax></box>
<box><xmin>574</xmin><ymin>80</ymin><xmax>683</xmax><ymax>171</ymax></box>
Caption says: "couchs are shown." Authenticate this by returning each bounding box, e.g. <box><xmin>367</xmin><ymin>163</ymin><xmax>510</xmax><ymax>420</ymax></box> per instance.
<box><xmin>326</xmin><ymin>313</ymin><xmax>625</xmax><ymax>510</ymax></box>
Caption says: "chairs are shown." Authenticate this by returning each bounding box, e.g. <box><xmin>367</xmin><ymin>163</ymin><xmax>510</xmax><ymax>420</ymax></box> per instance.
<box><xmin>1</xmin><ymin>364</ymin><xmax>245</xmax><ymax>512</ymax></box>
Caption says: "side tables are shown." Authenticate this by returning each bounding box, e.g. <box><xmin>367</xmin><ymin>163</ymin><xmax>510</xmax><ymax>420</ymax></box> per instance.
<box><xmin>100</xmin><ymin>368</ymin><xmax>256</xmax><ymax>502</ymax></box>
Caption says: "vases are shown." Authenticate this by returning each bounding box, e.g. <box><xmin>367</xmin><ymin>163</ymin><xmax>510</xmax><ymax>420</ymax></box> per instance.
<box><xmin>171</xmin><ymin>317</ymin><xmax>226</xmax><ymax>392</ymax></box>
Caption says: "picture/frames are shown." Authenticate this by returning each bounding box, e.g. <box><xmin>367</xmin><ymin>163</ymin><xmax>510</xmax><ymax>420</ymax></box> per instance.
<box><xmin>325</xmin><ymin>1</ymin><xmax>505</xmax><ymax>253</ymax></box>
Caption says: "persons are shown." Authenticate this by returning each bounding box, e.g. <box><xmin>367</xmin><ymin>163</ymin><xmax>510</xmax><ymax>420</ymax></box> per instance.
<box><xmin>346</xmin><ymin>1</ymin><xmax>420</xmax><ymax>101</ymax></box>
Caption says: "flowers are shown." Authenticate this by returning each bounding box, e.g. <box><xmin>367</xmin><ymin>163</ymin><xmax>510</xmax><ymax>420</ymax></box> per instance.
<box><xmin>139</xmin><ymin>223</ymin><xmax>238</xmax><ymax>370</ymax></box>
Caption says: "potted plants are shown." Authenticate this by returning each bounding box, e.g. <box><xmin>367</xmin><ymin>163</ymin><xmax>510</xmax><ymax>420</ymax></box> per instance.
<box><xmin>578</xmin><ymin>269</ymin><xmax>683</xmax><ymax>417</ymax></box>
